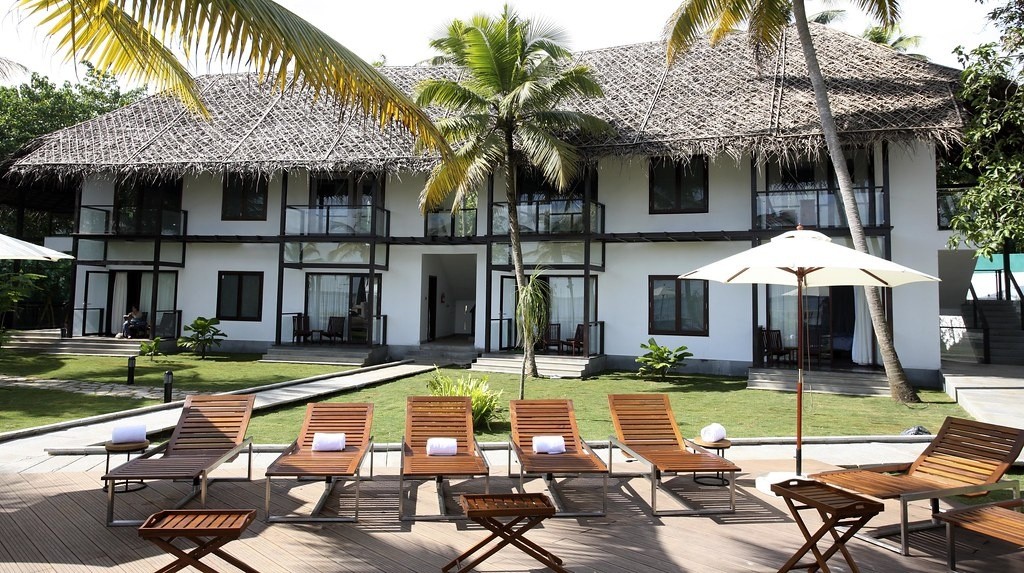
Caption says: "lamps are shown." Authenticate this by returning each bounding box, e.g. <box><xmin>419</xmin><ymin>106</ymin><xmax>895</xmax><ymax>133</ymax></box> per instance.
<box><xmin>126</xmin><ymin>356</ymin><xmax>136</xmax><ymax>384</ymax></box>
<box><xmin>164</xmin><ymin>370</ymin><xmax>173</xmax><ymax>402</ymax></box>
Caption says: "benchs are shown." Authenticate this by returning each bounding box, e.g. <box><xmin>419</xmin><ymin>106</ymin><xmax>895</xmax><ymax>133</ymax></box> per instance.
<box><xmin>769</xmin><ymin>477</ymin><xmax>886</xmax><ymax>573</ymax></box>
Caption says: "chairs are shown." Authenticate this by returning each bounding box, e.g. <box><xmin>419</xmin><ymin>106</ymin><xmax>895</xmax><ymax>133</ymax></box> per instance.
<box><xmin>122</xmin><ymin>312</ymin><xmax>148</xmax><ymax>338</ymax></box>
<box><xmin>605</xmin><ymin>392</ymin><xmax>743</xmax><ymax>518</ymax></box>
<box><xmin>543</xmin><ymin>323</ymin><xmax>561</xmax><ymax>353</ymax></box>
<box><xmin>503</xmin><ymin>397</ymin><xmax>612</xmax><ymax>520</ymax></box>
<box><xmin>560</xmin><ymin>324</ymin><xmax>592</xmax><ymax>355</ymax></box>
<box><xmin>762</xmin><ymin>329</ymin><xmax>788</xmax><ymax>367</ymax></box>
<box><xmin>103</xmin><ymin>439</ymin><xmax>151</xmax><ymax>494</ymax></box>
<box><xmin>321</xmin><ymin>315</ymin><xmax>346</xmax><ymax>345</ymax></box>
<box><xmin>98</xmin><ymin>392</ymin><xmax>258</xmax><ymax>529</ymax></box>
<box><xmin>395</xmin><ymin>394</ymin><xmax>493</xmax><ymax>525</ymax></box>
<box><xmin>156</xmin><ymin>312</ymin><xmax>175</xmax><ymax>336</ymax></box>
<box><xmin>262</xmin><ymin>400</ymin><xmax>378</xmax><ymax>525</ymax></box>
<box><xmin>803</xmin><ymin>415</ymin><xmax>1024</xmax><ymax>573</ymax></box>
<box><xmin>293</xmin><ymin>315</ymin><xmax>313</xmax><ymax>344</ymax></box>
<box><xmin>804</xmin><ymin>324</ymin><xmax>824</xmax><ymax>368</ymax></box>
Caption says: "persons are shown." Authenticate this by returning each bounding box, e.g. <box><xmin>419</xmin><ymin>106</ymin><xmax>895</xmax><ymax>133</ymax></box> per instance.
<box><xmin>123</xmin><ymin>305</ymin><xmax>143</xmax><ymax>339</ymax></box>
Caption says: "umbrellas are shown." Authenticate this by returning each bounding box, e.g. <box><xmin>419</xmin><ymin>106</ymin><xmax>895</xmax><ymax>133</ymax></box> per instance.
<box><xmin>677</xmin><ymin>225</ymin><xmax>943</xmax><ymax>476</ymax></box>
<box><xmin>0</xmin><ymin>233</ymin><xmax>76</xmax><ymax>262</ymax></box>
<box><xmin>654</xmin><ymin>284</ymin><xmax>675</xmax><ymax>321</ymax></box>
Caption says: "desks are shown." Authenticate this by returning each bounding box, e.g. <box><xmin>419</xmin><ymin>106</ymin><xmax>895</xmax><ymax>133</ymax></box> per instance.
<box><xmin>311</xmin><ymin>329</ymin><xmax>323</xmax><ymax>344</ymax></box>
<box><xmin>439</xmin><ymin>491</ymin><xmax>572</xmax><ymax>573</ymax></box>
<box><xmin>136</xmin><ymin>506</ymin><xmax>262</xmax><ymax>573</ymax></box>
<box><xmin>692</xmin><ymin>436</ymin><xmax>732</xmax><ymax>487</ymax></box>
<box><xmin>785</xmin><ymin>346</ymin><xmax>799</xmax><ymax>366</ymax></box>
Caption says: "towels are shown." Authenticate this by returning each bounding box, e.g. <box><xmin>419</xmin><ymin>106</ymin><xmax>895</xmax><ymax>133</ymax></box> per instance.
<box><xmin>311</xmin><ymin>432</ymin><xmax>346</xmax><ymax>451</ymax></box>
<box><xmin>111</xmin><ymin>425</ymin><xmax>147</xmax><ymax>444</ymax></box>
<box><xmin>531</xmin><ymin>435</ymin><xmax>566</xmax><ymax>454</ymax></box>
<box><xmin>426</xmin><ymin>437</ymin><xmax>458</xmax><ymax>457</ymax></box>
<box><xmin>700</xmin><ymin>422</ymin><xmax>726</xmax><ymax>443</ymax></box>
